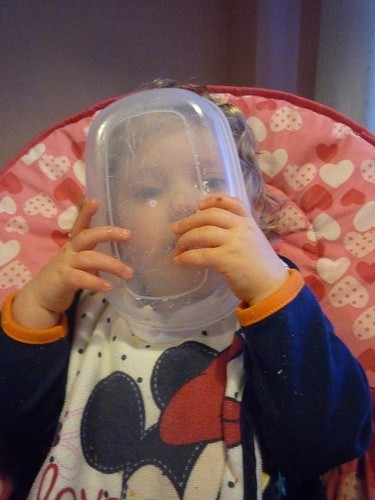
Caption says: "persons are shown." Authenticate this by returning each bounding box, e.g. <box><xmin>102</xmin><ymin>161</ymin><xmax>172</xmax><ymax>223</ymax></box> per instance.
<box><xmin>0</xmin><ymin>79</ymin><xmax>375</xmax><ymax>500</ymax></box>
